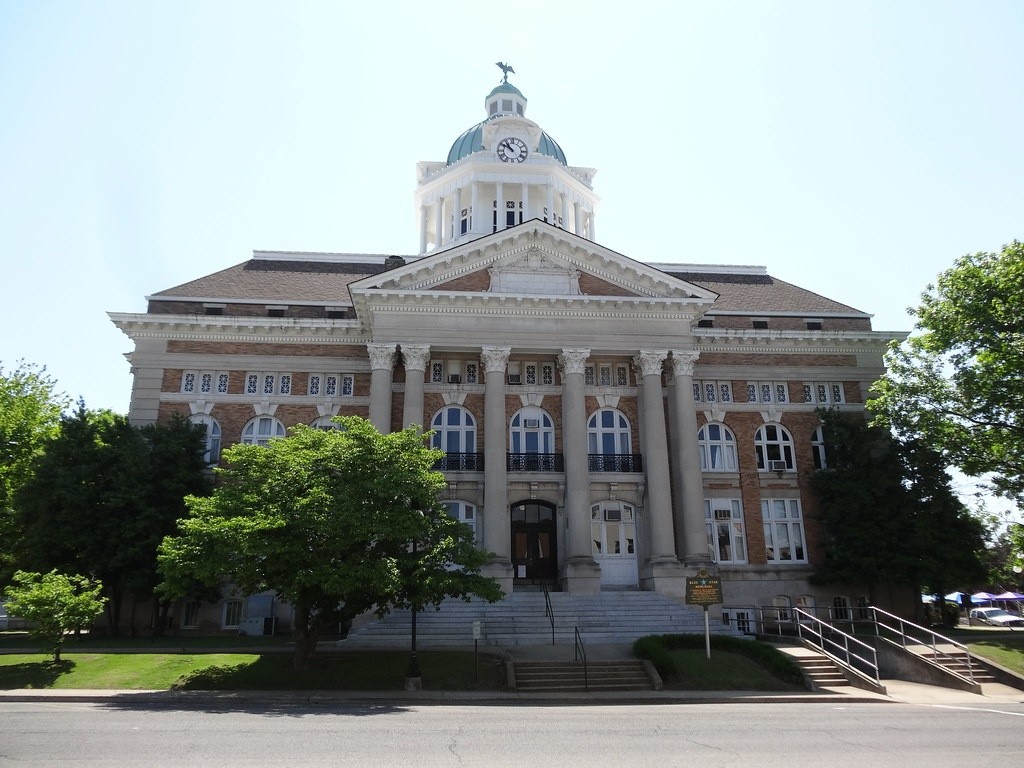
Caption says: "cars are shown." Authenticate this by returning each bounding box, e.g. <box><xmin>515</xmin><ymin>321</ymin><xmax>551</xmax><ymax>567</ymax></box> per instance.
<box><xmin>970</xmin><ymin>607</ymin><xmax>1024</xmax><ymax>627</ymax></box>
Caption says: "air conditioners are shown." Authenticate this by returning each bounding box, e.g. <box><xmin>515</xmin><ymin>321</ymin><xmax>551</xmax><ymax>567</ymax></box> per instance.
<box><xmin>768</xmin><ymin>460</ymin><xmax>787</xmax><ymax>471</ymax></box>
<box><xmin>603</xmin><ymin>509</ymin><xmax>622</xmax><ymax>521</ymax></box>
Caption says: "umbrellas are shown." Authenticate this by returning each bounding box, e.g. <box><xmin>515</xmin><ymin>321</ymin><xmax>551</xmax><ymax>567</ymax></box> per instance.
<box><xmin>921</xmin><ymin>592</ymin><xmax>1024</xmax><ymax>610</ymax></box>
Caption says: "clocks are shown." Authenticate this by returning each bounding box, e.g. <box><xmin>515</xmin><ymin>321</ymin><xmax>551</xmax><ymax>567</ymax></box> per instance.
<box><xmin>497</xmin><ymin>137</ymin><xmax>530</xmax><ymax>163</ymax></box>
